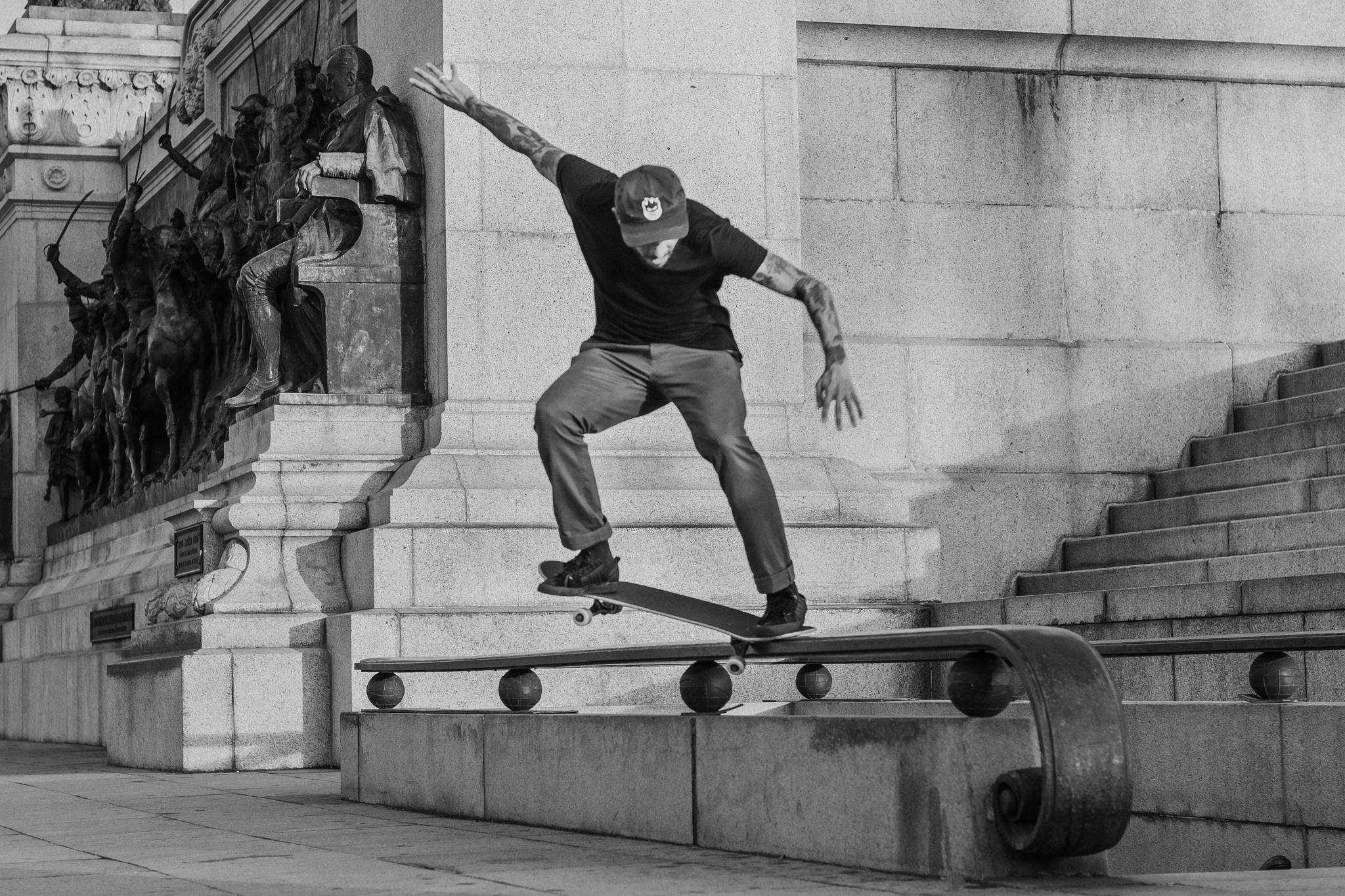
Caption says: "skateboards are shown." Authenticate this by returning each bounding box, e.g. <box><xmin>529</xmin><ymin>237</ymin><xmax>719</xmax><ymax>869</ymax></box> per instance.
<box><xmin>538</xmin><ymin>561</ymin><xmax>817</xmax><ymax>675</ymax></box>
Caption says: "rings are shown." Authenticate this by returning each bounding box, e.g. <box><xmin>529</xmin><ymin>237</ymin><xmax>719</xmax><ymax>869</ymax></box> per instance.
<box><xmin>439</xmin><ymin>76</ymin><xmax>445</xmax><ymax>80</ymax></box>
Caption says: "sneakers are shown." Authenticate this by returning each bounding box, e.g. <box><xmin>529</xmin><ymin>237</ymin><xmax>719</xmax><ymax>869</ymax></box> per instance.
<box><xmin>538</xmin><ymin>550</ymin><xmax>622</xmax><ymax>597</ymax></box>
<box><xmin>756</xmin><ymin>590</ymin><xmax>807</xmax><ymax>638</ymax></box>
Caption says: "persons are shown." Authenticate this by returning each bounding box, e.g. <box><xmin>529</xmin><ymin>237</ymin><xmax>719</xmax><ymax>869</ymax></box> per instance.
<box><xmin>224</xmin><ymin>44</ymin><xmax>425</xmax><ymax>408</ymax></box>
<box><xmin>408</xmin><ymin>60</ymin><xmax>867</xmax><ymax>638</ymax></box>
<box><xmin>33</xmin><ymin>93</ymin><xmax>309</xmax><ymax>520</ymax></box>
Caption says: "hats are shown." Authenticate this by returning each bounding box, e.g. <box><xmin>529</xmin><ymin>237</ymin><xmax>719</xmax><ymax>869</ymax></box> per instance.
<box><xmin>615</xmin><ymin>165</ymin><xmax>689</xmax><ymax>248</ymax></box>
